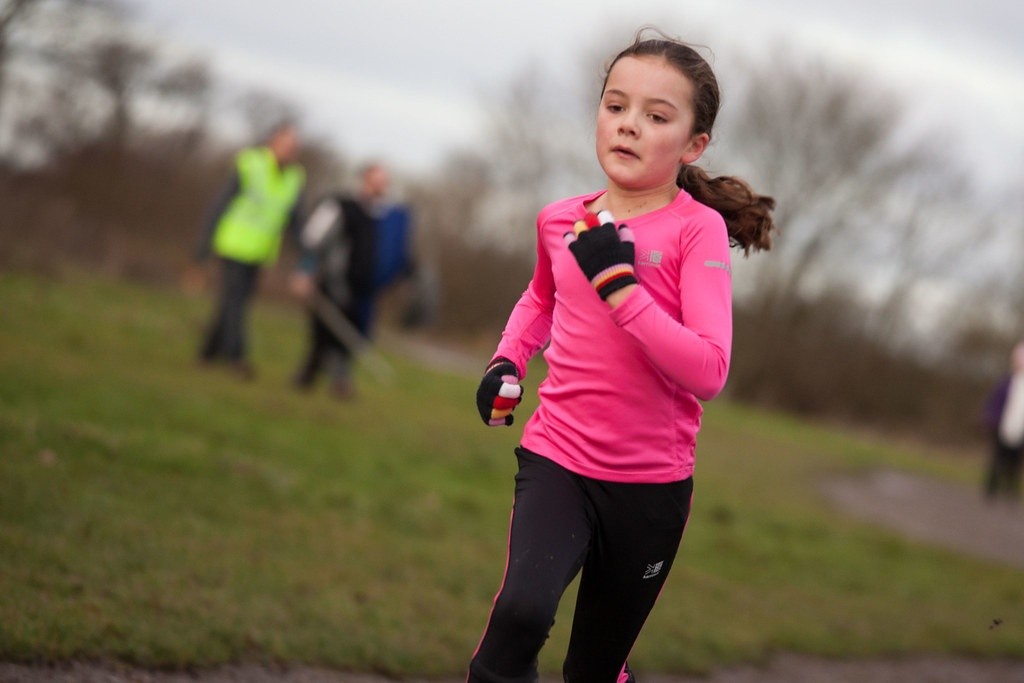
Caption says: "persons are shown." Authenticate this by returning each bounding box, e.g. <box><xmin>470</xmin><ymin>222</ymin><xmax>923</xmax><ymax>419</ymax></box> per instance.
<box><xmin>197</xmin><ymin>121</ymin><xmax>315</xmax><ymax>373</ymax></box>
<box><xmin>466</xmin><ymin>24</ymin><xmax>776</xmax><ymax>683</ymax></box>
<box><xmin>299</xmin><ymin>162</ymin><xmax>417</xmax><ymax>395</ymax></box>
<box><xmin>983</xmin><ymin>341</ymin><xmax>1024</xmax><ymax>500</ymax></box>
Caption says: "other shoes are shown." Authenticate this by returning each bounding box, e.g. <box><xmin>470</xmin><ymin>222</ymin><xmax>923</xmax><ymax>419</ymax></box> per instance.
<box><xmin>616</xmin><ymin>662</ymin><xmax>634</xmax><ymax>682</ymax></box>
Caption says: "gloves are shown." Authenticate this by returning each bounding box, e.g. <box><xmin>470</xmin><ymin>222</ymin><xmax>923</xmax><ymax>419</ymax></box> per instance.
<box><xmin>563</xmin><ymin>209</ymin><xmax>638</xmax><ymax>300</ymax></box>
<box><xmin>476</xmin><ymin>358</ymin><xmax>524</xmax><ymax>426</ymax></box>
